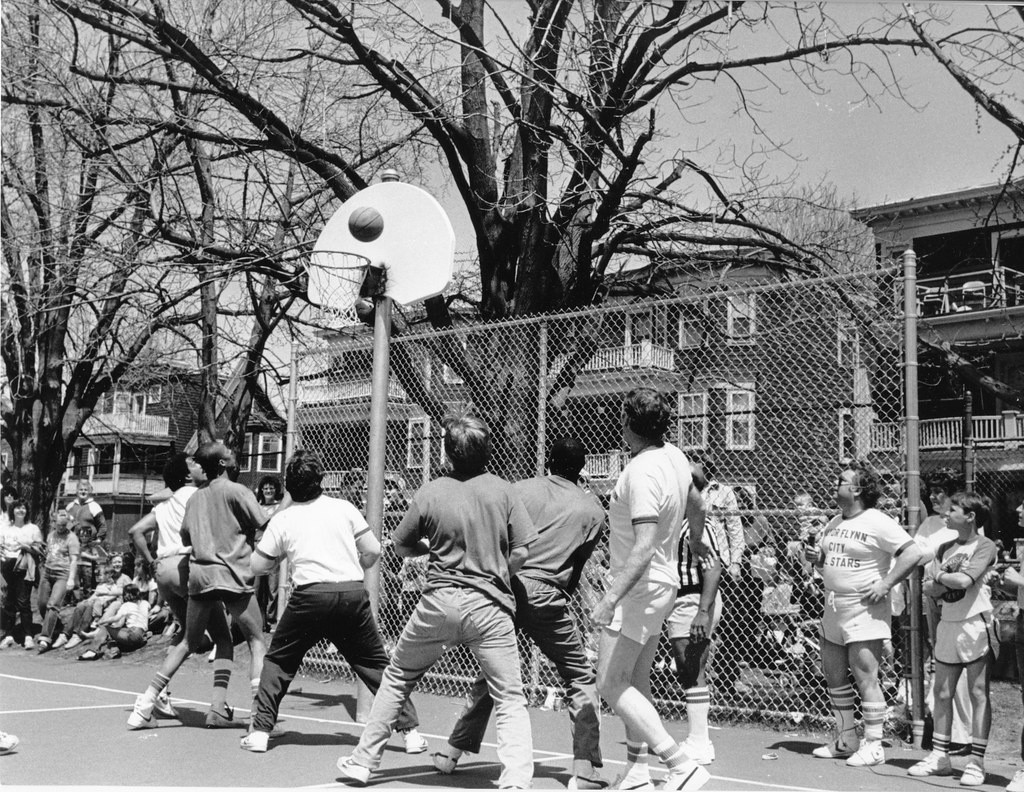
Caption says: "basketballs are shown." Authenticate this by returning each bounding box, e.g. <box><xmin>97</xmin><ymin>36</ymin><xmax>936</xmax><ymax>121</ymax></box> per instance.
<box><xmin>349</xmin><ymin>206</ymin><xmax>383</xmax><ymax>241</ymax></box>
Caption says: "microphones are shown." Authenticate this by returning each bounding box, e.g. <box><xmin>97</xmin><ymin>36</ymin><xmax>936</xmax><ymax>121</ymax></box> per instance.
<box><xmin>809</xmin><ymin>529</ymin><xmax>818</xmax><ymax>568</ymax></box>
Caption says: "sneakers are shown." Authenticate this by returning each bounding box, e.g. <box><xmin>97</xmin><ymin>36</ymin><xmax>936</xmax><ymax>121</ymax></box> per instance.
<box><xmin>813</xmin><ymin>735</ymin><xmax>860</xmax><ymax>757</ymax></box>
<box><xmin>908</xmin><ymin>752</ymin><xmax>953</xmax><ymax>776</ymax></box>
<box><xmin>663</xmin><ymin>762</ymin><xmax>711</xmax><ymax>791</ymax></box>
<box><xmin>961</xmin><ymin>760</ymin><xmax>986</xmax><ymax>786</ymax></box>
<box><xmin>601</xmin><ymin>773</ymin><xmax>655</xmax><ymax>790</ymax></box>
<box><xmin>568</xmin><ymin>767</ymin><xmax>612</xmax><ymax>790</ymax></box>
<box><xmin>248</xmin><ymin>720</ymin><xmax>286</xmax><ymax>738</ymax></box>
<box><xmin>1006</xmin><ymin>769</ymin><xmax>1024</xmax><ymax>792</ymax></box>
<box><xmin>0</xmin><ymin>731</ymin><xmax>19</xmax><ymax>756</ymax></box>
<box><xmin>127</xmin><ymin>710</ymin><xmax>159</xmax><ymax>728</ymax></box>
<box><xmin>240</xmin><ymin>733</ymin><xmax>269</xmax><ymax>752</ymax></box>
<box><xmin>134</xmin><ymin>691</ymin><xmax>180</xmax><ymax>716</ymax></box>
<box><xmin>432</xmin><ymin>749</ymin><xmax>458</xmax><ymax>774</ymax></box>
<box><xmin>846</xmin><ymin>740</ymin><xmax>885</xmax><ymax>766</ymax></box>
<box><xmin>205</xmin><ymin>702</ymin><xmax>244</xmax><ymax>727</ymax></box>
<box><xmin>406</xmin><ymin>737</ymin><xmax>428</xmax><ymax>753</ymax></box>
<box><xmin>337</xmin><ymin>757</ymin><xmax>371</xmax><ymax>785</ymax></box>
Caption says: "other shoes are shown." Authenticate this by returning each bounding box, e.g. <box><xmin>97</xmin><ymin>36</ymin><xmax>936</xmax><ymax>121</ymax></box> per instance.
<box><xmin>38</xmin><ymin>641</ymin><xmax>48</xmax><ymax>652</ymax></box>
<box><xmin>659</xmin><ymin>736</ymin><xmax>715</xmax><ymax>765</ymax></box>
<box><xmin>52</xmin><ymin>633</ymin><xmax>68</xmax><ymax>648</ymax></box>
<box><xmin>64</xmin><ymin>633</ymin><xmax>82</xmax><ymax>649</ymax></box>
<box><xmin>76</xmin><ymin>653</ymin><xmax>99</xmax><ymax>660</ymax></box>
<box><xmin>24</xmin><ymin>636</ymin><xmax>35</xmax><ymax>649</ymax></box>
<box><xmin>0</xmin><ymin>636</ymin><xmax>15</xmax><ymax>649</ymax></box>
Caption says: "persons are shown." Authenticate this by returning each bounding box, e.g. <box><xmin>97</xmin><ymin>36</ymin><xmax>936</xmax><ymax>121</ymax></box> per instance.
<box><xmin>804</xmin><ymin>465</ymin><xmax>922</xmax><ymax>766</ymax></box>
<box><xmin>239</xmin><ymin>448</ymin><xmax>431</xmax><ymax>754</ymax></box>
<box><xmin>336</xmin><ymin>412</ymin><xmax>539</xmax><ymax>791</ymax></box>
<box><xmin>908</xmin><ymin>488</ymin><xmax>1000</xmax><ymax>787</ymax></box>
<box><xmin>0</xmin><ymin>388</ymin><xmax>1024</xmax><ymax>788</ymax></box>
<box><xmin>590</xmin><ymin>386</ymin><xmax>719</xmax><ymax>787</ymax></box>
<box><xmin>431</xmin><ymin>438</ymin><xmax>611</xmax><ymax>788</ymax></box>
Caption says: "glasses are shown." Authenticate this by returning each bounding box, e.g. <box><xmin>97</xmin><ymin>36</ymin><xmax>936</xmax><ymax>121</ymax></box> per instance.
<box><xmin>838</xmin><ymin>475</ymin><xmax>855</xmax><ymax>486</ymax></box>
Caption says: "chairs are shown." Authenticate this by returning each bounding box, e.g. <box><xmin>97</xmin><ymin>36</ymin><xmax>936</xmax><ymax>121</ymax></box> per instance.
<box><xmin>922</xmin><ymin>286</ymin><xmax>948</xmax><ymax>314</ymax></box>
<box><xmin>961</xmin><ymin>281</ymin><xmax>987</xmax><ymax>309</ymax></box>
